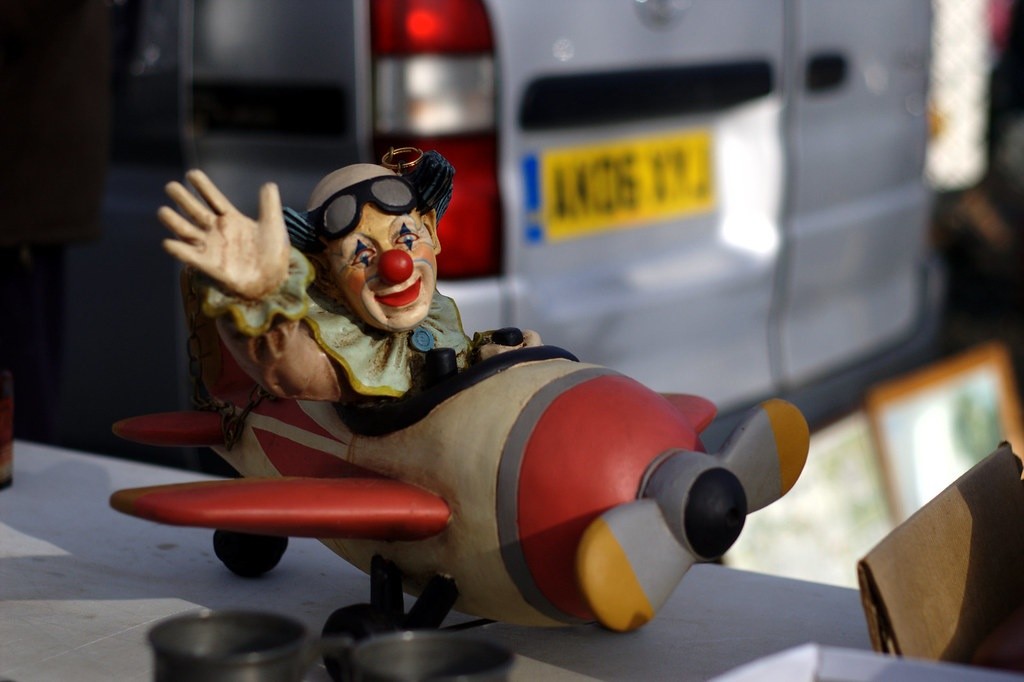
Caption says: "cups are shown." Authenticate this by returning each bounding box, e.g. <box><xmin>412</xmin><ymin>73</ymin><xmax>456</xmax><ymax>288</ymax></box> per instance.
<box><xmin>149</xmin><ymin>610</ymin><xmax>352</xmax><ymax>682</ymax></box>
<box><xmin>355</xmin><ymin>630</ymin><xmax>515</xmax><ymax>682</ymax></box>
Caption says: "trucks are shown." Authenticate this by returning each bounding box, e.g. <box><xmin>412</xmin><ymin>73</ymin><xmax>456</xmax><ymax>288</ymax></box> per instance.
<box><xmin>0</xmin><ymin>0</ymin><xmax>940</xmax><ymax>479</ymax></box>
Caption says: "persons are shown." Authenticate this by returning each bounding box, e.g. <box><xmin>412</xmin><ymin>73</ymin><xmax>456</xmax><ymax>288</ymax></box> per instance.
<box><xmin>157</xmin><ymin>145</ymin><xmax>544</xmax><ymax>406</ymax></box>
<box><xmin>0</xmin><ymin>1</ymin><xmax>113</xmax><ymax>446</ymax></box>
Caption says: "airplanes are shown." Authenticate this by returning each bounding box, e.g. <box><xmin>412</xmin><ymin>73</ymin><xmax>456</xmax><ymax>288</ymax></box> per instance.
<box><xmin>110</xmin><ymin>263</ymin><xmax>809</xmax><ymax>633</ymax></box>
